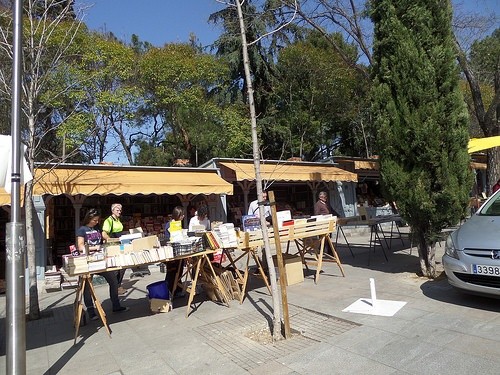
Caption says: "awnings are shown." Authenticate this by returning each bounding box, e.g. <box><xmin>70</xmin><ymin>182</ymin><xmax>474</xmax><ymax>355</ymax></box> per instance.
<box><xmin>32</xmin><ymin>168</ymin><xmax>233</xmax><ymax>196</ymax></box>
<box><xmin>217</xmin><ymin>161</ymin><xmax>358</xmax><ymax>182</ymax></box>
<box><xmin>0</xmin><ymin>185</ymin><xmax>25</xmax><ymax>207</ymax></box>
<box><xmin>336</xmin><ymin>158</ymin><xmax>381</xmax><ymax>170</ymax></box>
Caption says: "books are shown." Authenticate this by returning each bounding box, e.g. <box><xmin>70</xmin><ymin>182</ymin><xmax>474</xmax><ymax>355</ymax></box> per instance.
<box><xmin>242</xmin><ymin>215</ymin><xmax>262</xmax><ymax>242</ymax></box>
<box><xmin>167</xmin><ymin>220</ymin><xmax>237</xmax><ymax>255</ymax></box>
<box><xmin>62</xmin><ymin>233</ymin><xmax>174</xmax><ymax>274</ymax></box>
<box><xmin>283</xmin><ymin>215</ymin><xmax>333</xmax><ymax>236</ymax></box>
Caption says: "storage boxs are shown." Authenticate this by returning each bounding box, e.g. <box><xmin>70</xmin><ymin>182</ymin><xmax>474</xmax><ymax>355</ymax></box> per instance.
<box><xmin>272</xmin><ymin>253</ymin><xmax>304</xmax><ymax>286</ymax></box>
<box><xmin>150</xmin><ymin>297</ymin><xmax>171</xmax><ymax>312</ymax></box>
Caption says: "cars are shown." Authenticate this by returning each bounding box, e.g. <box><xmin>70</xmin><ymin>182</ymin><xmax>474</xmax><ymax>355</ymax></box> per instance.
<box><xmin>441</xmin><ymin>187</ymin><xmax>500</xmax><ymax>299</ymax></box>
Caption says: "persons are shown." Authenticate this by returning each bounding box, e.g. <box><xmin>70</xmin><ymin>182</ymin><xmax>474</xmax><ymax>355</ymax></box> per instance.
<box><xmin>165</xmin><ymin>206</ymin><xmax>186</xmax><ymax>296</ymax></box>
<box><xmin>188</xmin><ymin>207</ymin><xmax>211</xmax><ymax>280</ymax></box>
<box><xmin>102</xmin><ymin>203</ymin><xmax>127</xmax><ymax>294</ymax></box>
<box><xmin>248</xmin><ymin>190</ymin><xmax>272</xmax><ymax>278</ymax></box>
<box><xmin>492</xmin><ymin>179</ymin><xmax>500</xmax><ymax>194</ymax></box>
<box><xmin>76</xmin><ymin>209</ymin><xmax>130</xmax><ymax>320</ymax></box>
<box><xmin>481</xmin><ymin>188</ymin><xmax>487</xmax><ymax>199</ymax></box>
<box><xmin>314</xmin><ymin>191</ymin><xmax>340</xmax><ymax>258</ymax></box>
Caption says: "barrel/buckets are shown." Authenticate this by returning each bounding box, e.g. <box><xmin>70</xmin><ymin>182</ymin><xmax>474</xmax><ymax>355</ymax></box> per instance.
<box><xmin>146</xmin><ymin>281</ymin><xmax>169</xmax><ymax>299</ymax></box>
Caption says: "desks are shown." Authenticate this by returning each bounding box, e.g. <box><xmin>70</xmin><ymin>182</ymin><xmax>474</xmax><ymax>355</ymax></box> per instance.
<box><xmin>63</xmin><ymin>247</ymin><xmax>230</xmax><ymax>347</ymax></box>
<box><xmin>220</xmin><ymin>218</ymin><xmax>345</xmax><ymax>305</ymax></box>
<box><xmin>335</xmin><ymin>215</ymin><xmax>404</xmax><ymax>262</ymax></box>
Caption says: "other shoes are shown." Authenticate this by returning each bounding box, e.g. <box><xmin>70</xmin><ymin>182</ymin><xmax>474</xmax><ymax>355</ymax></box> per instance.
<box><xmin>113</xmin><ymin>306</ymin><xmax>126</xmax><ymax>312</ymax></box>
<box><xmin>117</xmin><ymin>287</ymin><xmax>128</xmax><ymax>295</ymax></box>
<box><xmin>88</xmin><ymin>315</ymin><xmax>99</xmax><ymax>321</ymax></box>
